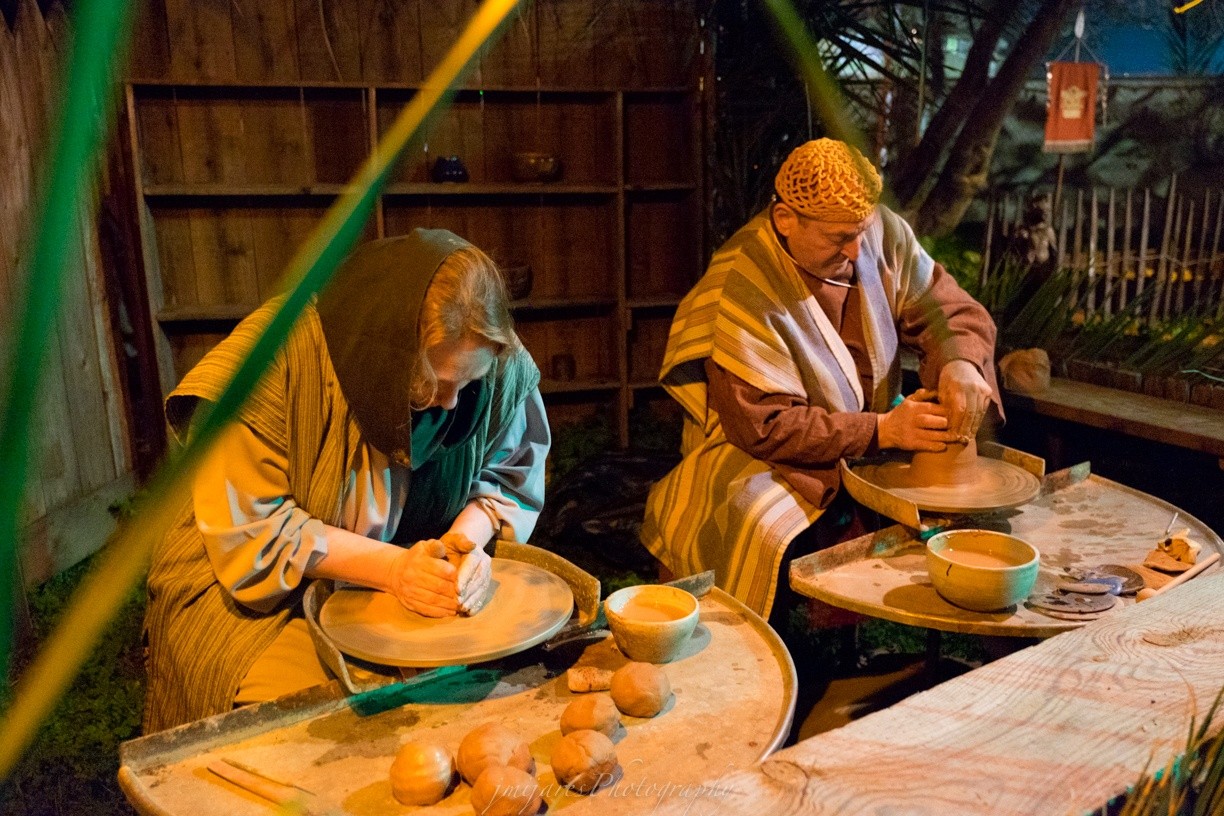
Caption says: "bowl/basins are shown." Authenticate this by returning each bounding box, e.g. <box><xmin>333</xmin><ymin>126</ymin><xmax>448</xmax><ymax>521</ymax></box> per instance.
<box><xmin>606</xmin><ymin>584</ymin><xmax>701</xmax><ymax>663</ymax></box>
<box><xmin>510</xmin><ymin>151</ymin><xmax>562</xmax><ymax>183</ymax></box>
<box><xmin>496</xmin><ymin>261</ymin><xmax>532</xmax><ymax>300</ymax></box>
<box><xmin>926</xmin><ymin>529</ymin><xmax>1041</xmax><ymax>611</ymax></box>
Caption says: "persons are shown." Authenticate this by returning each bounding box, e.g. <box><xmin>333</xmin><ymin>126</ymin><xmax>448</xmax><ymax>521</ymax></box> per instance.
<box><xmin>668</xmin><ymin>139</ymin><xmax>1004</xmax><ymax>627</ymax></box>
<box><xmin>146</xmin><ymin>227</ymin><xmax>552</xmax><ymax>706</ymax></box>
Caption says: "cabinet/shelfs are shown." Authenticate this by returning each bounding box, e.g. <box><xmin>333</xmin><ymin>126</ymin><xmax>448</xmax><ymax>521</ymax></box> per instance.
<box><xmin>114</xmin><ymin>71</ymin><xmax>713</xmax><ymax>470</ymax></box>
